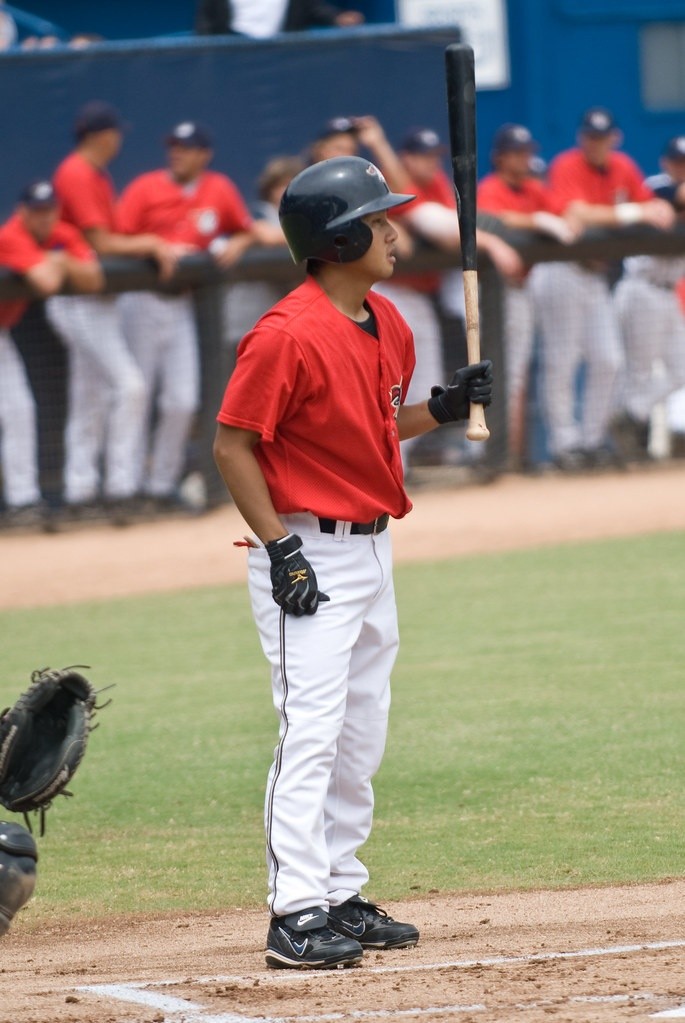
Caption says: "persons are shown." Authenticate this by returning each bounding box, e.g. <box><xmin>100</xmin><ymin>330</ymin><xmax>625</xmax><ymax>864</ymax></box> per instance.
<box><xmin>45</xmin><ymin>99</ymin><xmax>166</xmax><ymax>515</ymax></box>
<box><xmin>307</xmin><ymin>113</ymin><xmax>423</xmax><ymax>218</ymax></box>
<box><xmin>105</xmin><ymin>116</ymin><xmax>258</xmax><ymax>528</ymax></box>
<box><xmin>386</xmin><ymin>128</ymin><xmax>527</xmax><ymax>481</ymax></box>
<box><xmin>225</xmin><ymin>154</ymin><xmax>312</xmax><ymax>361</ymax></box>
<box><xmin>470</xmin><ymin>123</ymin><xmax>585</xmax><ymax>474</ymax></box>
<box><xmin>529</xmin><ymin>110</ymin><xmax>675</xmax><ymax>469</ymax></box>
<box><xmin>609</xmin><ymin>135</ymin><xmax>685</xmax><ymax>472</ymax></box>
<box><xmin>0</xmin><ymin>183</ymin><xmax>103</xmax><ymax>527</ymax></box>
<box><xmin>209</xmin><ymin>158</ymin><xmax>497</xmax><ymax>971</ymax></box>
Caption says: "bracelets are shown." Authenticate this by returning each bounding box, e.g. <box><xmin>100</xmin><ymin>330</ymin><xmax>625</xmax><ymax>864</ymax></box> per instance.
<box><xmin>615</xmin><ymin>203</ymin><xmax>642</xmax><ymax>227</ymax></box>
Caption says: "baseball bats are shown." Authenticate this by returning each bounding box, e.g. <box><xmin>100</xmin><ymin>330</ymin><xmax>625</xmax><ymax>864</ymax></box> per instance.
<box><xmin>445</xmin><ymin>42</ymin><xmax>491</xmax><ymax>441</ymax></box>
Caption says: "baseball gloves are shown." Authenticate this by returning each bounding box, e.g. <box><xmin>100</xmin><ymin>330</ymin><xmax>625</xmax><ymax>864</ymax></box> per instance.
<box><xmin>0</xmin><ymin>670</ymin><xmax>96</xmax><ymax>813</ymax></box>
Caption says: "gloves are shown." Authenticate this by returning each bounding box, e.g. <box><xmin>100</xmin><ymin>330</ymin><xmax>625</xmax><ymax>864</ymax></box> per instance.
<box><xmin>263</xmin><ymin>533</ymin><xmax>330</xmax><ymax>617</ymax></box>
<box><xmin>426</xmin><ymin>361</ymin><xmax>494</xmax><ymax>425</ymax></box>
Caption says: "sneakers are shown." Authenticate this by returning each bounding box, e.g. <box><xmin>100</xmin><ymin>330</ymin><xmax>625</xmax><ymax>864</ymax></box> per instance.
<box><xmin>264</xmin><ymin>907</ymin><xmax>363</xmax><ymax>970</ymax></box>
<box><xmin>326</xmin><ymin>894</ymin><xmax>419</xmax><ymax>949</ymax></box>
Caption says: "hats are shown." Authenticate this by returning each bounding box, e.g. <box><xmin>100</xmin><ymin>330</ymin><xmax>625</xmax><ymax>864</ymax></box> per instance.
<box><xmin>315</xmin><ymin>115</ymin><xmax>359</xmax><ymax>140</ymax></box>
<box><xmin>496</xmin><ymin>123</ymin><xmax>534</xmax><ymax>152</ymax></box>
<box><xmin>170</xmin><ymin>120</ymin><xmax>212</xmax><ymax>149</ymax></box>
<box><xmin>580</xmin><ymin>107</ymin><xmax>621</xmax><ymax>137</ymax></box>
<box><xmin>23</xmin><ymin>181</ymin><xmax>58</xmax><ymax>210</ymax></box>
<box><xmin>665</xmin><ymin>135</ymin><xmax>685</xmax><ymax>162</ymax></box>
<box><xmin>400</xmin><ymin>126</ymin><xmax>440</xmax><ymax>153</ymax></box>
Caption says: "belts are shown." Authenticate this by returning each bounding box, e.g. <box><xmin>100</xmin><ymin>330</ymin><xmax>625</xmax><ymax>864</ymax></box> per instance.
<box><xmin>318</xmin><ymin>515</ymin><xmax>390</xmax><ymax>535</ymax></box>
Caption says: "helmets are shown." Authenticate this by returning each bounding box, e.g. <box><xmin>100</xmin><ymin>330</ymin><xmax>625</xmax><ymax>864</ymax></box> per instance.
<box><xmin>278</xmin><ymin>156</ymin><xmax>416</xmax><ymax>264</ymax></box>
